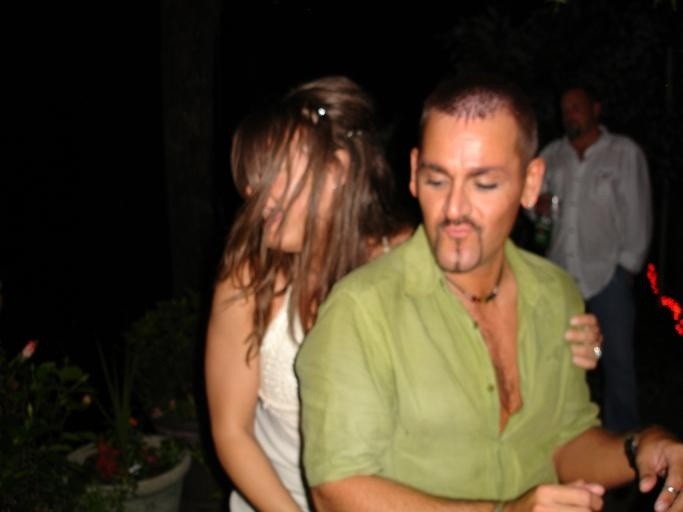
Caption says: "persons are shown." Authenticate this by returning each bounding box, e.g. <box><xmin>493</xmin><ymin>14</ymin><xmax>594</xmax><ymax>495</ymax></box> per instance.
<box><xmin>204</xmin><ymin>76</ymin><xmax>603</xmax><ymax>512</ymax></box>
<box><xmin>297</xmin><ymin>76</ymin><xmax>683</xmax><ymax>512</ymax></box>
<box><xmin>528</xmin><ymin>80</ymin><xmax>652</xmax><ymax>433</ymax></box>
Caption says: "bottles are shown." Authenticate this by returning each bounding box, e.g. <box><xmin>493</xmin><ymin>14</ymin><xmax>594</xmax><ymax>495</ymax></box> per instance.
<box><xmin>532</xmin><ymin>189</ymin><xmax>553</xmax><ymax>252</ymax></box>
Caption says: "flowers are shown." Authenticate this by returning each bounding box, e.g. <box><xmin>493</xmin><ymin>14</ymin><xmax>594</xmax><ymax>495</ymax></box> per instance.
<box><xmin>0</xmin><ymin>294</ymin><xmax>227</xmax><ymax>512</ymax></box>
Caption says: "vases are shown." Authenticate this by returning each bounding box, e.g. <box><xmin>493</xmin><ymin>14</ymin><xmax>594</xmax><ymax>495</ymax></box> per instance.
<box><xmin>63</xmin><ymin>434</ymin><xmax>193</xmax><ymax>511</ymax></box>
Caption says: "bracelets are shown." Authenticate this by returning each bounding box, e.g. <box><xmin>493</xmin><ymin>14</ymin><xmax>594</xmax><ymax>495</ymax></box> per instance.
<box><xmin>624</xmin><ymin>434</ymin><xmax>637</xmax><ymax>469</ymax></box>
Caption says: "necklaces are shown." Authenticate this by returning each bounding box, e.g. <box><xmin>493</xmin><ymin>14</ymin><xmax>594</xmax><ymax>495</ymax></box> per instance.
<box><xmin>445</xmin><ymin>258</ymin><xmax>505</xmax><ymax>303</ymax></box>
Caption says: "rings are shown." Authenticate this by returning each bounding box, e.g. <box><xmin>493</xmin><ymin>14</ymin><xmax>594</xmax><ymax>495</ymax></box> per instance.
<box><xmin>665</xmin><ymin>486</ymin><xmax>675</xmax><ymax>493</ymax></box>
<box><xmin>594</xmin><ymin>347</ymin><xmax>601</xmax><ymax>358</ymax></box>
